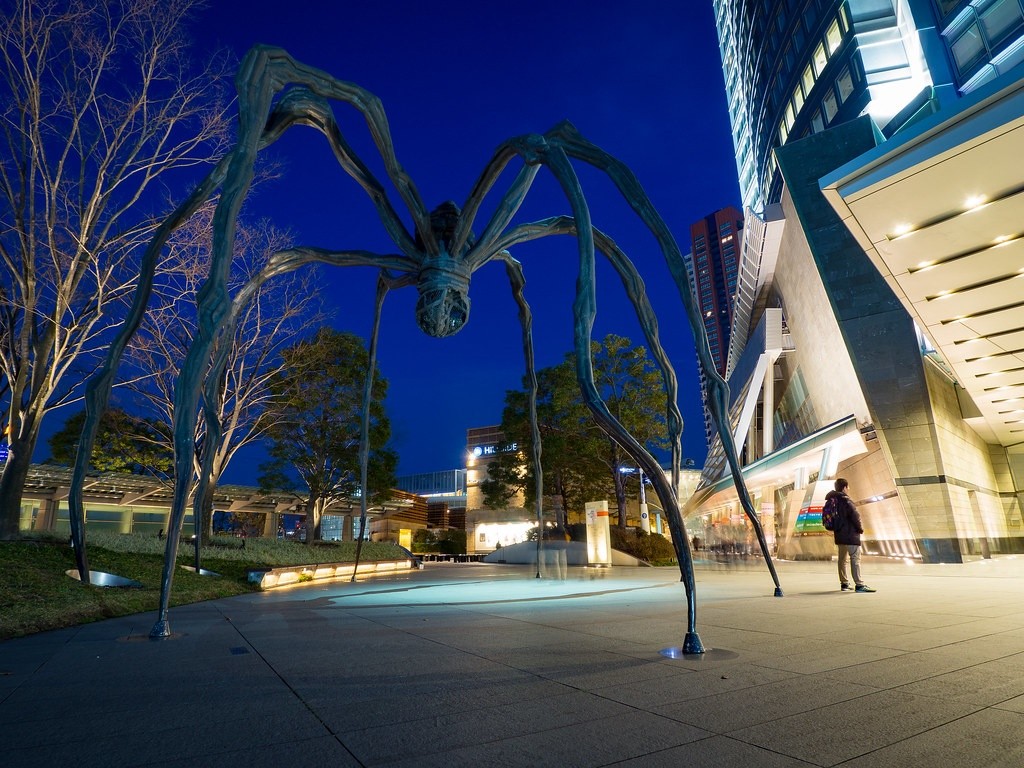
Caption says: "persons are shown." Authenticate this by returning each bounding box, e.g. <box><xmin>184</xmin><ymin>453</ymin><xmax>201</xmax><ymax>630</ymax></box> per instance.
<box><xmin>834</xmin><ymin>479</ymin><xmax>876</xmax><ymax>592</ymax></box>
<box><xmin>692</xmin><ymin>535</ymin><xmax>699</xmax><ymax>551</ymax></box>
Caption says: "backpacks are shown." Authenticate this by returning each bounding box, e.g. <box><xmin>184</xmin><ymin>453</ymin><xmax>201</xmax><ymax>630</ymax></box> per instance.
<box><xmin>822</xmin><ymin>497</ymin><xmax>843</xmax><ymax>530</ymax></box>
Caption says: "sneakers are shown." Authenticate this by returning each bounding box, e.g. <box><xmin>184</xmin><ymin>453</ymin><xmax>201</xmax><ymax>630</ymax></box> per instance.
<box><xmin>841</xmin><ymin>584</ymin><xmax>855</xmax><ymax>591</ymax></box>
<box><xmin>856</xmin><ymin>586</ymin><xmax>876</xmax><ymax>592</ymax></box>
